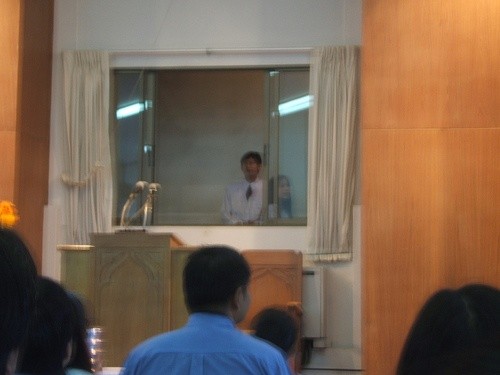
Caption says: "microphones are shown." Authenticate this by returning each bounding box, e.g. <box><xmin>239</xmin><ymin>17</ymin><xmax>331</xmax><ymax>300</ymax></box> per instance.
<box><xmin>114</xmin><ymin>181</ymin><xmax>160</xmax><ymax>232</ymax></box>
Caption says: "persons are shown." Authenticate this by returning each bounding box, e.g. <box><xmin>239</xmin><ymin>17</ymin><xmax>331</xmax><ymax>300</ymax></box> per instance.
<box><xmin>396</xmin><ymin>284</ymin><xmax>500</xmax><ymax>375</ymax></box>
<box><xmin>118</xmin><ymin>245</ymin><xmax>296</xmax><ymax>375</ymax></box>
<box><xmin>222</xmin><ymin>151</ymin><xmax>292</xmax><ymax>225</ymax></box>
<box><xmin>0</xmin><ymin>227</ymin><xmax>94</xmax><ymax>375</ymax></box>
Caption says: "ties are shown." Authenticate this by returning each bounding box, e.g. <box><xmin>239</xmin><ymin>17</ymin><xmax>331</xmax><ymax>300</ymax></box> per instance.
<box><xmin>246</xmin><ymin>185</ymin><xmax>252</xmax><ymax>199</ymax></box>
